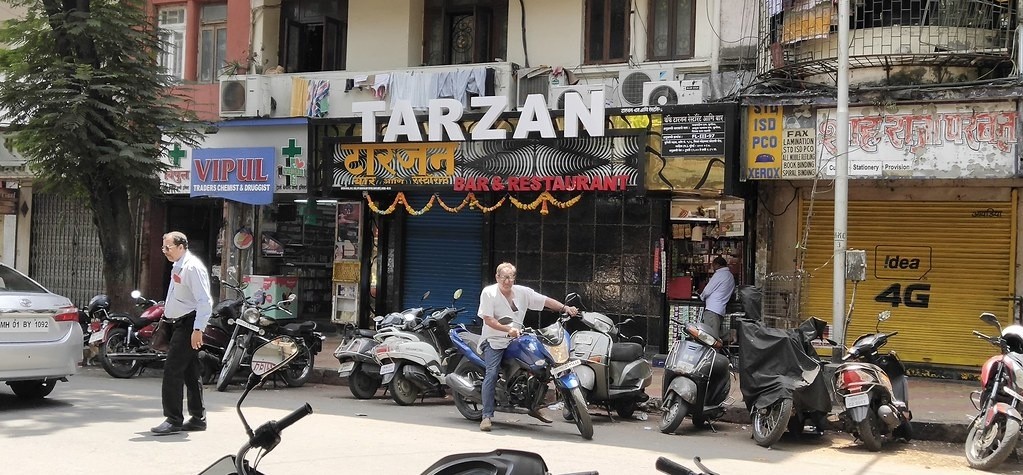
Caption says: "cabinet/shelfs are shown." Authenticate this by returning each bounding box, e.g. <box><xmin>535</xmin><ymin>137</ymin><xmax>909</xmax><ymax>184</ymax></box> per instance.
<box><xmin>276</xmin><ymin>204</ymin><xmax>337</xmax><ymax>247</ymax></box>
<box><xmin>286</xmin><ymin>262</ymin><xmax>334</xmax><ymax>313</ymax></box>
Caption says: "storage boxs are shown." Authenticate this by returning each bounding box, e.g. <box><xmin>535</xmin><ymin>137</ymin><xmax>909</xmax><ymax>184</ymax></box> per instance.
<box><xmin>88</xmin><ymin>295</ymin><xmax>111</xmax><ymax>318</ymax></box>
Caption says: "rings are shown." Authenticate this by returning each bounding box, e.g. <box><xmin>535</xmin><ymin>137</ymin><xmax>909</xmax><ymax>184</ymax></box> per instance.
<box><xmin>197</xmin><ymin>342</ymin><xmax>200</xmax><ymax>344</ymax></box>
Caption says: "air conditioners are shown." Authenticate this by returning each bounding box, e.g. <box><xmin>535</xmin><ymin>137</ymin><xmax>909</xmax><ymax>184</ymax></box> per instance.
<box><xmin>641</xmin><ymin>80</ymin><xmax>703</xmax><ymax>105</ymax></box>
<box><xmin>619</xmin><ymin>67</ymin><xmax>679</xmax><ymax>108</ymax></box>
<box><xmin>516</xmin><ymin>72</ymin><xmax>570</xmax><ymax>110</ymax></box>
<box><xmin>549</xmin><ymin>84</ymin><xmax>614</xmax><ymax>109</ymax></box>
<box><xmin>219</xmin><ymin>74</ymin><xmax>272</xmax><ymax>118</ymax></box>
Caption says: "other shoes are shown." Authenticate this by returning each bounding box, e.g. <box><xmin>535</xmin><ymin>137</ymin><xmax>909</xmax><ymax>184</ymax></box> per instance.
<box><xmin>528</xmin><ymin>409</ymin><xmax>553</xmax><ymax>423</ymax></box>
<box><xmin>480</xmin><ymin>418</ymin><xmax>491</xmax><ymax>431</ymax></box>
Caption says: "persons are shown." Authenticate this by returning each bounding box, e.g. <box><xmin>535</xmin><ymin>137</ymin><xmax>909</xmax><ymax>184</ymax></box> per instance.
<box><xmin>477</xmin><ymin>262</ymin><xmax>579</xmax><ymax>431</ymax></box>
<box><xmin>150</xmin><ymin>232</ymin><xmax>214</xmax><ymax>434</ymax></box>
<box><xmin>699</xmin><ymin>257</ymin><xmax>735</xmax><ymax>335</ymax></box>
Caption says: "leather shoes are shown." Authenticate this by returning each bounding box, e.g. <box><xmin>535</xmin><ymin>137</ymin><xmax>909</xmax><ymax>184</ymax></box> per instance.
<box><xmin>181</xmin><ymin>420</ymin><xmax>206</xmax><ymax>431</ymax></box>
<box><xmin>152</xmin><ymin>420</ymin><xmax>183</xmax><ymax>433</ymax></box>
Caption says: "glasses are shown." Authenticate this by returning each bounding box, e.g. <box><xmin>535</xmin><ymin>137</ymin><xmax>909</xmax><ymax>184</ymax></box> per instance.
<box><xmin>497</xmin><ymin>274</ymin><xmax>515</xmax><ymax>281</ymax></box>
<box><xmin>161</xmin><ymin>244</ymin><xmax>178</xmax><ymax>252</ymax></box>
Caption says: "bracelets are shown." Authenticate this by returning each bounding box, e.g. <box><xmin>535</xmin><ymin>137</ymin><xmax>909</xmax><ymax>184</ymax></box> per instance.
<box><xmin>194</xmin><ymin>328</ymin><xmax>200</xmax><ymax>331</ymax></box>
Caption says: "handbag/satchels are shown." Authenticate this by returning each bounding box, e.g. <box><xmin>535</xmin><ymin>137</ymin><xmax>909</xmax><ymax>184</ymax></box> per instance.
<box><xmin>150</xmin><ymin>316</ymin><xmax>172</xmax><ymax>352</ymax></box>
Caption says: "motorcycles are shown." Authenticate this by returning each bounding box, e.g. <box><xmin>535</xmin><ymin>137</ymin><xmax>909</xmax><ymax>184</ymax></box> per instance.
<box><xmin>442</xmin><ymin>292</ymin><xmax>597</xmax><ymax>441</ymax></box>
<box><xmin>88</xmin><ymin>290</ymin><xmax>179</xmax><ymax>379</ymax></box>
<box><xmin>194</xmin><ymin>266</ymin><xmax>327</xmax><ymax>392</ymax></box>
<box><xmin>735</xmin><ymin>314</ymin><xmax>827</xmax><ymax>447</ymax></box>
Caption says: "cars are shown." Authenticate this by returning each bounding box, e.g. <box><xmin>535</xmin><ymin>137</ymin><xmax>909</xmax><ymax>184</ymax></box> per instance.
<box><xmin>0</xmin><ymin>264</ymin><xmax>87</xmax><ymax>399</ymax></box>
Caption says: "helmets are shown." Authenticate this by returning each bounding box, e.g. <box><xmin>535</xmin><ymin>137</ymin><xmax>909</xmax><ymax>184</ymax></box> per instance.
<box><xmin>1002</xmin><ymin>325</ymin><xmax>1023</xmax><ymax>353</ymax></box>
<box><xmin>89</xmin><ymin>293</ymin><xmax>110</xmax><ymax>318</ymax></box>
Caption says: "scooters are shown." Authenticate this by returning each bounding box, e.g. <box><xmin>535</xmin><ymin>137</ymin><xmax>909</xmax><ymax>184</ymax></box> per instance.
<box><xmin>964</xmin><ymin>311</ymin><xmax>1023</xmax><ymax>471</ymax></box>
<box><xmin>562</xmin><ymin>294</ymin><xmax>653</xmax><ymax>423</ymax></box>
<box><xmin>825</xmin><ymin>309</ymin><xmax>914</xmax><ymax>454</ymax></box>
<box><xmin>333</xmin><ymin>287</ymin><xmax>471</xmax><ymax>407</ymax></box>
<box><xmin>190</xmin><ymin>338</ymin><xmax>724</xmax><ymax>475</ymax></box>
<box><xmin>657</xmin><ymin>316</ymin><xmax>738</xmax><ymax>434</ymax></box>
